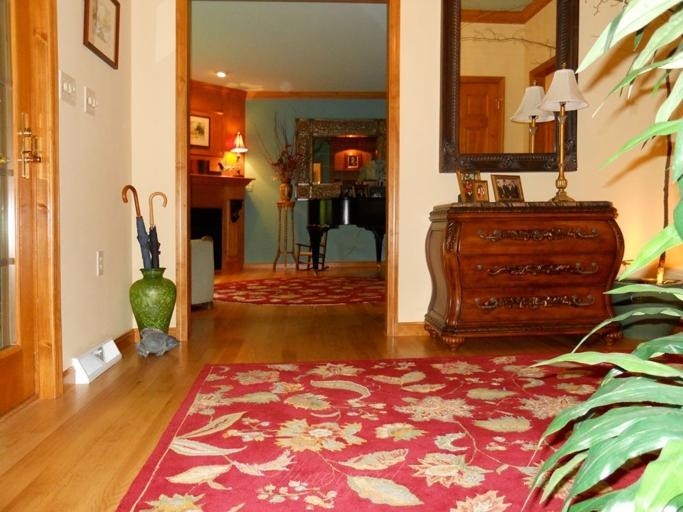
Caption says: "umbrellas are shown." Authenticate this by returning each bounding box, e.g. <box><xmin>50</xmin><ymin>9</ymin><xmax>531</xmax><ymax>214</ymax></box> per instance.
<box><xmin>147</xmin><ymin>191</ymin><xmax>167</xmax><ymax>271</ymax></box>
<box><xmin>121</xmin><ymin>185</ymin><xmax>151</xmax><ymax>269</ymax></box>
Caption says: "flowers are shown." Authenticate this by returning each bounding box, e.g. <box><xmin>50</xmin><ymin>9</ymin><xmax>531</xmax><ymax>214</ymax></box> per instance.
<box><xmin>248</xmin><ymin>102</ymin><xmax>316</xmax><ymax>183</ymax></box>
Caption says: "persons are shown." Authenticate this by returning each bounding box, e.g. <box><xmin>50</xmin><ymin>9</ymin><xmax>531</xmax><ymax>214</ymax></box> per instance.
<box><xmin>463</xmin><ymin>177</ymin><xmax>521</xmax><ymax>203</ymax></box>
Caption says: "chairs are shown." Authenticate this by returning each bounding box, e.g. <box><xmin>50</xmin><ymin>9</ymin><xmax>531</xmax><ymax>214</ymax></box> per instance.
<box><xmin>296</xmin><ymin>224</ymin><xmax>329</xmax><ymax>271</ymax></box>
<box><xmin>189</xmin><ymin>235</ymin><xmax>217</xmax><ymax>311</ymax></box>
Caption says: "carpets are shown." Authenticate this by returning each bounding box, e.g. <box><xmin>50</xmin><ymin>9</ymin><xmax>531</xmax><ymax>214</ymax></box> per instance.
<box><xmin>115</xmin><ymin>340</ymin><xmax>683</xmax><ymax>512</ymax></box>
<box><xmin>214</xmin><ymin>274</ymin><xmax>388</xmax><ymax>308</ymax></box>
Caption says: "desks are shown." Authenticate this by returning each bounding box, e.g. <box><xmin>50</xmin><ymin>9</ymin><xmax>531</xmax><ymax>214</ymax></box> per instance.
<box><xmin>305</xmin><ymin>197</ymin><xmax>387</xmax><ymax>269</ymax></box>
<box><xmin>189</xmin><ymin>173</ymin><xmax>257</xmax><ymax>274</ymax></box>
<box><xmin>272</xmin><ymin>201</ymin><xmax>297</xmax><ymax>271</ymax></box>
<box><xmin>425</xmin><ymin>200</ymin><xmax>627</xmax><ymax>351</ymax></box>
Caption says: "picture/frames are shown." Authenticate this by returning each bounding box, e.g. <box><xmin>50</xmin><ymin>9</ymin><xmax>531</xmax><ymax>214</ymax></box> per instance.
<box><xmin>472</xmin><ymin>181</ymin><xmax>490</xmax><ymax>203</ymax></box>
<box><xmin>344</xmin><ymin>151</ymin><xmax>363</xmax><ymax>169</ymax></box>
<box><xmin>190</xmin><ymin>116</ymin><xmax>211</xmax><ymax>149</ymax></box>
<box><xmin>312</xmin><ymin>160</ymin><xmax>322</xmax><ymax>184</ymax></box>
<box><xmin>455</xmin><ymin>168</ymin><xmax>482</xmax><ymax>203</ymax></box>
<box><xmin>340</xmin><ymin>180</ymin><xmax>377</xmax><ymax>199</ymax></box>
<box><xmin>490</xmin><ymin>172</ymin><xmax>524</xmax><ymax>203</ymax></box>
<box><xmin>82</xmin><ymin>1</ymin><xmax>121</xmax><ymax>71</ymax></box>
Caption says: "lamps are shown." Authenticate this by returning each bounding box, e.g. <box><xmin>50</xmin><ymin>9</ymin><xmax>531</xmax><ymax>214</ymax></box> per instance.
<box><xmin>231</xmin><ymin>131</ymin><xmax>249</xmax><ymax>178</ymax></box>
<box><xmin>509</xmin><ymin>86</ymin><xmax>556</xmax><ymax>153</ymax></box>
<box><xmin>536</xmin><ymin>62</ymin><xmax>589</xmax><ymax>201</ymax></box>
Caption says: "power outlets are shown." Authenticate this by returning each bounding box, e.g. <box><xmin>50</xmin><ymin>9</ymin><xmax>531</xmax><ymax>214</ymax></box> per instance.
<box><xmin>96</xmin><ymin>250</ymin><xmax>105</xmax><ymax>275</ymax></box>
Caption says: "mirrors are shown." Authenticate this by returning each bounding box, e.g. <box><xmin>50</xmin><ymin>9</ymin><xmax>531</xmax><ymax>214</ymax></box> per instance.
<box><xmin>438</xmin><ymin>1</ymin><xmax>580</xmax><ymax>173</ymax></box>
<box><xmin>295</xmin><ymin>115</ymin><xmax>387</xmax><ymax>200</ymax></box>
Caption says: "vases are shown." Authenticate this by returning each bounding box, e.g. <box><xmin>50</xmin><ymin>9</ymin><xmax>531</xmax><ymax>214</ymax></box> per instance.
<box><xmin>128</xmin><ymin>266</ymin><xmax>177</xmax><ymax>342</ymax></box>
<box><xmin>279</xmin><ymin>178</ymin><xmax>293</xmax><ymax>201</ymax></box>
